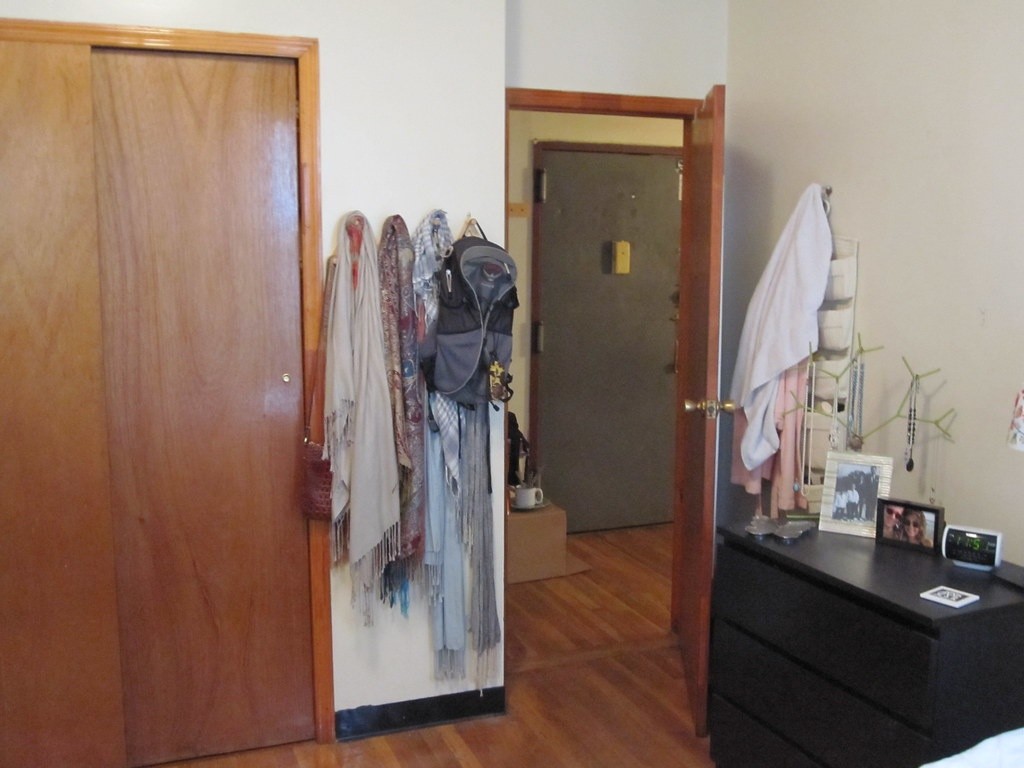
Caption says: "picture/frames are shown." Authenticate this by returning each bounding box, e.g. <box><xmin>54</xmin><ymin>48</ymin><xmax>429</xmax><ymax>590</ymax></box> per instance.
<box><xmin>876</xmin><ymin>496</ymin><xmax>944</xmax><ymax>556</ymax></box>
<box><xmin>818</xmin><ymin>451</ymin><xmax>893</xmax><ymax>539</ymax></box>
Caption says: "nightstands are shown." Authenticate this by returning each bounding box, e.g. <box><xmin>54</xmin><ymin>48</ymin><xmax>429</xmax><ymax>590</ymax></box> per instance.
<box><xmin>711</xmin><ymin>515</ymin><xmax>1024</xmax><ymax>768</ymax></box>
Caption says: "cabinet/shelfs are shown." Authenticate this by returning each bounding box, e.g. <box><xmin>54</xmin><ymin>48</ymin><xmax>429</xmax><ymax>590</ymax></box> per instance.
<box><xmin>0</xmin><ymin>19</ymin><xmax>329</xmax><ymax>767</ymax></box>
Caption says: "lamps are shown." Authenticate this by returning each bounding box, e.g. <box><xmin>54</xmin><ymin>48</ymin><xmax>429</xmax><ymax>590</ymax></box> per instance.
<box><xmin>1005</xmin><ymin>390</ymin><xmax>1024</xmax><ymax>450</ymax></box>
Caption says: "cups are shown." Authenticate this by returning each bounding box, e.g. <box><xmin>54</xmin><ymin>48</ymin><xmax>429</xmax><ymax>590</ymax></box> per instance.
<box><xmin>515</xmin><ymin>484</ymin><xmax>543</xmax><ymax>506</ymax></box>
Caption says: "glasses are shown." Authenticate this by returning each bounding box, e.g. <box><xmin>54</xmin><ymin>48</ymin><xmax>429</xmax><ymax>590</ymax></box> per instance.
<box><xmin>886</xmin><ymin>507</ymin><xmax>904</xmax><ymax>522</ymax></box>
<box><xmin>904</xmin><ymin>519</ymin><xmax>919</xmax><ymax>527</ymax></box>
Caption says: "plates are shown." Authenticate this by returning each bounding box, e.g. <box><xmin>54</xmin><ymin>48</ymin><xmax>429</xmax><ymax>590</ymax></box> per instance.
<box><xmin>510</xmin><ymin>497</ymin><xmax>551</xmax><ymax>510</ymax></box>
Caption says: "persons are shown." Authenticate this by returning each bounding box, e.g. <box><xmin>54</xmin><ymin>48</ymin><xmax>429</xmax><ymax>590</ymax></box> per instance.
<box><xmin>882</xmin><ymin>504</ymin><xmax>905</xmax><ymax>540</ymax></box>
<box><xmin>895</xmin><ymin>507</ymin><xmax>932</xmax><ymax>547</ymax></box>
<box><xmin>831</xmin><ymin>465</ymin><xmax>880</xmax><ymax>521</ymax></box>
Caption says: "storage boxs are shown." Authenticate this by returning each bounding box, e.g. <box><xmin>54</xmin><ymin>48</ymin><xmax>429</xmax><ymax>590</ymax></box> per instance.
<box><xmin>507</xmin><ymin>500</ymin><xmax>593</xmax><ymax>584</ymax></box>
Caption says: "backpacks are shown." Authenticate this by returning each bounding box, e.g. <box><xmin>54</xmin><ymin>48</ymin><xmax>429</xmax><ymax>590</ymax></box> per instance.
<box><xmin>506</xmin><ymin>412</ymin><xmax>530</xmax><ymax>486</ymax></box>
<box><xmin>418</xmin><ymin>219</ymin><xmax>520</xmax><ymax>407</ymax></box>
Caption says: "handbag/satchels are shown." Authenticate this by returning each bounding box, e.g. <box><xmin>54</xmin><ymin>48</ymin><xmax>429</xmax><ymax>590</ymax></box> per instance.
<box><xmin>302</xmin><ymin>441</ymin><xmax>348</xmax><ymax>526</ymax></box>
